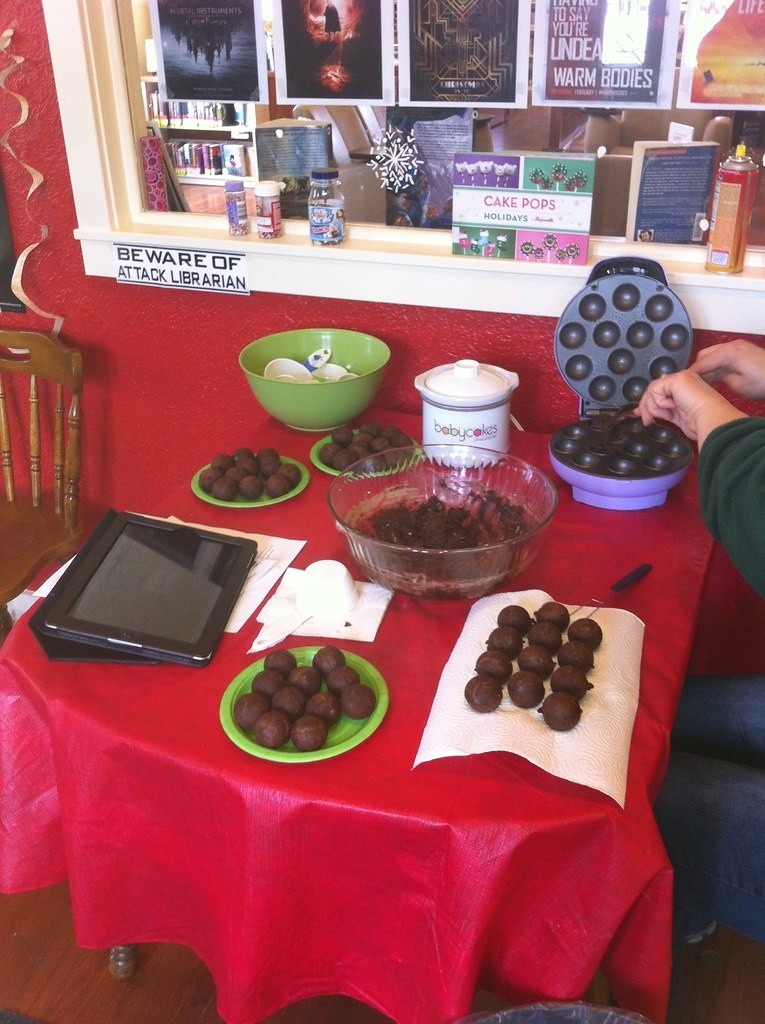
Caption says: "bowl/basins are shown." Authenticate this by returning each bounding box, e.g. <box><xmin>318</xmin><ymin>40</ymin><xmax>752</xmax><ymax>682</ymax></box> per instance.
<box><xmin>239</xmin><ymin>328</ymin><xmax>391</xmax><ymax>432</ymax></box>
<box><xmin>327</xmin><ymin>443</ymin><xmax>556</xmax><ymax>599</ymax></box>
<box><xmin>296</xmin><ymin>560</ymin><xmax>360</xmax><ymax>620</ymax></box>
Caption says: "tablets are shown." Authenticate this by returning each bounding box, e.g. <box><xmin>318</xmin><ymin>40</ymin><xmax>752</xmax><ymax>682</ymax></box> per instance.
<box><xmin>27</xmin><ymin>507</ymin><xmax>259</xmax><ymax>669</ymax></box>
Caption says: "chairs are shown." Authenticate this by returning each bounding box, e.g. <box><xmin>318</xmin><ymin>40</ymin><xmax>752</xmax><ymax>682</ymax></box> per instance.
<box><xmin>0</xmin><ymin>326</ymin><xmax>88</xmax><ymax>649</ymax></box>
<box><xmin>292</xmin><ymin>105</ymin><xmax>732</xmax><ymax>236</ymax></box>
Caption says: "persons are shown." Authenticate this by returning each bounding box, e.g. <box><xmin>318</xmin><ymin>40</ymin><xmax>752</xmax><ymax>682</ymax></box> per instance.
<box><xmin>638</xmin><ymin>340</ymin><xmax>765</xmax><ymax>944</ymax></box>
<box><xmin>639</xmin><ymin>231</ymin><xmax>652</xmax><ymax>242</ymax></box>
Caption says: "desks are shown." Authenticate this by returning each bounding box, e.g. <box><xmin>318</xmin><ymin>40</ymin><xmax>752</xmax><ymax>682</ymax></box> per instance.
<box><xmin>0</xmin><ymin>405</ymin><xmax>716</xmax><ymax>1024</ymax></box>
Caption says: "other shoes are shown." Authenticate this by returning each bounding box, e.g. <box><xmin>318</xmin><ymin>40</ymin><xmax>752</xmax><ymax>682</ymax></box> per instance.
<box><xmin>672</xmin><ymin>904</ymin><xmax>715</xmax><ymax>944</ymax></box>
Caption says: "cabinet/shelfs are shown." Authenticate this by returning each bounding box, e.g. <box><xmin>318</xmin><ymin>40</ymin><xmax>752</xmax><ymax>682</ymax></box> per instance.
<box><xmin>138</xmin><ymin>72</ymin><xmax>297</xmax><ymax>187</ymax></box>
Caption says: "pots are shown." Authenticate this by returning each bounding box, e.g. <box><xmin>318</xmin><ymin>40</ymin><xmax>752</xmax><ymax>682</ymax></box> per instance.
<box><xmin>548</xmin><ymin>257</ymin><xmax>696</xmax><ymax>512</ymax></box>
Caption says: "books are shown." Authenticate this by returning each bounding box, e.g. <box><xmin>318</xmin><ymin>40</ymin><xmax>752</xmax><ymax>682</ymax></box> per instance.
<box><xmin>384</xmin><ymin>107</ymin><xmax>476</xmax><ymax>231</ymax></box>
<box><xmin>452</xmin><ymin>149</ymin><xmax>596</xmax><ymax>266</ymax></box>
<box><xmin>626</xmin><ymin>141</ymin><xmax>723</xmax><ymax>243</ymax></box>
<box><xmin>148</xmin><ymin>90</ymin><xmax>255</xmax><ymax>177</ymax></box>
<box><xmin>255</xmin><ymin>118</ymin><xmax>332</xmax><ymax>219</ymax></box>
<box><xmin>224</xmin><ymin>144</ymin><xmax>247</xmax><ymax>176</ymax></box>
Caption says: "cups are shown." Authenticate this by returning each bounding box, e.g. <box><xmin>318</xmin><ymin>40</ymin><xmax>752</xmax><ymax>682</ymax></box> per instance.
<box><xmin>415</xmin><ymin>360</ymin><xmax>519</xmax><ymax>469</ymax></box>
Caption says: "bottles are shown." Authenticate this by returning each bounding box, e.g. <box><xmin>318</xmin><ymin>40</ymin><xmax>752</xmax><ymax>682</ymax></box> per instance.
<box><xmin>308</xmin><ymin>168</ymin><xmax>346</xmax><ymax>245</ymax></box>
<box><xmin>255</xmin><ymin>180</ymin><xmax>283</xmax><ymax>239</ymax></box>
<box><xmin>705</xmin><ymin>144</ymin><xmax>760</xmax><ymax>275</ymax></box>
<box><xmin>224</xmin><ymin>180</ymin><xmax>249</xmax><ymax>236</ymax></box>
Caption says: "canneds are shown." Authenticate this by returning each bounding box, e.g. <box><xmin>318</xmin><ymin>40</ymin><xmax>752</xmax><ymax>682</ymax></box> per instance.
<box><xmin>225</xmin><ymin>181</ymin><xmax>286</xmax><ymax>238</ymax></box>
<box><xmin>307</xmin><ymin>168</ymin><xmax>347</xmax><ymax>246</ymax></box>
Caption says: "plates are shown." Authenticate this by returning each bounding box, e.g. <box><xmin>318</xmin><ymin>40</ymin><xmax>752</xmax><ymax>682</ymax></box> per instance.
<box><xmin>219</xmin><ymin>646</ymin><xmax>389</xmax><ymax>764</ymax></box>
<box><xmin>309</xmin><ymin>429</ymin><xmax>422</xmax><ymax>478</ymax></box>
<box><xmin>191</xmin><ymin>453</ymin><xmax>310</xmax><ymax>508</ymax></box>
